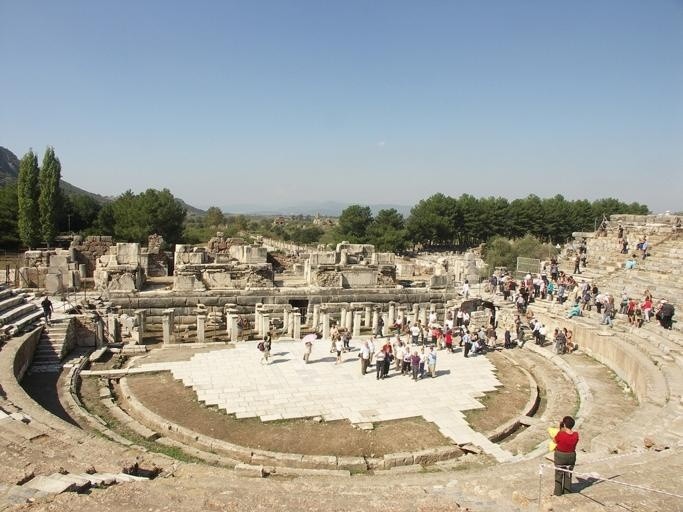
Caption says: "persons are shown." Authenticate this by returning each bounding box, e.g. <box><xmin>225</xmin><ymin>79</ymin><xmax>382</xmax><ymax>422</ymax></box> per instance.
<box><xmin>616</xmin><ymin>224</ymin><xmax>647</xmax><ymax>252</ymax></box>
<box><xmin>459</xmin><ymin>252</ymin><xmax>675</xmax><ymax>331</ymax></box>
<box><xmin>551</xmin><ymin>415</ymin><xmax>579</xmax><ymax>499</ymax></box>
<box><xmin>41</xmin><ymin>295</ymin><xmax>53</xmax><ymax>324</ymax></box>
<box><xmin>330</xmin><ymin>302</ymin><xmax>572</xmax><ymax>382</ymax></box>
<box><xmin>260</xmin><ymin>332</ymin><xmax>272</xmax><ymax>365</ymax></box>
<box><xmin>303</xmin><ymin>342</ymin><xmax>312</xmax><ymax>364</ymax></box>
<box><xmin>578</xmin><ymin>237</ymin><xmax>587</xmax><ymax>256</ymax></box>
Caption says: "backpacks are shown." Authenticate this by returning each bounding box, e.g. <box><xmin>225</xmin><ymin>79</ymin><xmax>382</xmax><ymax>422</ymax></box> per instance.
<box><xmin>257</xmin><ymin>342</ymin><xmax>264</xmax><ymax>351</ymax></box>
